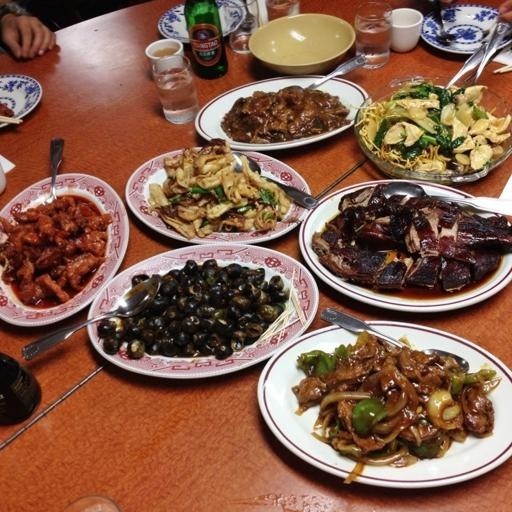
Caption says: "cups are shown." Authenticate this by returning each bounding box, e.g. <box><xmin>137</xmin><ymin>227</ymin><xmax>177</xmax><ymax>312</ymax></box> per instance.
<box><xmin>265</xmin><ymin>1</ymin><xmax>300</xmax><ymax>21</ymax></box>
<box><xmin>152</xmin><ymin>55</ymin><xmax>200</xmax><ymax>125</ymax></box>
<box><xmin>222</xmin><ymin>1</ymin><xmax>259</xmax><ymax>54</ymax></box>
<box><xmin>355</xmin><ymin>2</ymin><xmax>392</xmax><ymax>70</ymax></box>
<box><xmin>145</xmin><ymin>38</ymin><xmax>184</xmax><ymax>81</ymax></box>
<box><xmin>384</xmin><ymin>8</ymin><xmax>423</xmax><ymax>54</ymax></box>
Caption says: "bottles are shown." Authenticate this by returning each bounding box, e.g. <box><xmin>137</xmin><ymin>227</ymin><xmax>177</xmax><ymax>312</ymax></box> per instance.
<box><xmin>185</xmin><ymin>1</ymin><xmax>228</xmax><ymax>80</ymax></box>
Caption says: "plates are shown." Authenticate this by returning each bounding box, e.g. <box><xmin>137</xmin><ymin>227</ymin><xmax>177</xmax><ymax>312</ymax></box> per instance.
<box><xmin>421</xmin><ymin>5</ymin><xmax>511</xmax><ymax>55</ymax></box>
<box><xmin>124</xmin><ymin>146</ymin><xmax>311</xmax><ymax>244</ymax></box>
<box><xmin>298</xmin><ymin>179</ymin><xmax>512</xmax><ymax>314</ymax></box>
<box><xmin>193</xmin><ymin>76</ymin><xmax>372</xmax><ymax>151</ymax></box>
<box><xmin>0</xmin><ymin>174</ymin><xmax>131</xmax><ymax>326</ymax></box>
<box><xmin>0</xmin><ymin>74</ymin><xmax>43</xmax><ymax>128</ymax></box>
<box><xmin>251</xmin><ymin>320</ymin><xmax>511</xmax><ymax>488</ymax></box>
<box><xmin>158</xmin><ymin>0</ymin><xmax>246</xmax><ymax>43</ymax></box>
<box><xmin>87</xmin><ymin>243</ymin><xmax>320</xmax><ymax>379</ymax></box>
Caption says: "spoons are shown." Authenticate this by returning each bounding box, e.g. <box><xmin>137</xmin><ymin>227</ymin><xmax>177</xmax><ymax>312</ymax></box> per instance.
<box><xmin>320</xmin><ymin>307</ymin><xmax>469</xmax><ymax>373</ymax></box>
<box><xmin>425</xmin><ymin>0</ymin><xmax>457</xmax><ymax>45</ymax></box>
<box><xmin>382</xmin><ymin>183</ymin><xmax>511</xmax><ymax>216</ymax></box>
<box><xmin>21</xmin><ymin>275</ymin><xmax>161</xmax><ymax>361</ymax></box>
<box><xmin>232</xmin><ymin>152</ymin><xmax>319</xmax><ymax>211</ymax></box>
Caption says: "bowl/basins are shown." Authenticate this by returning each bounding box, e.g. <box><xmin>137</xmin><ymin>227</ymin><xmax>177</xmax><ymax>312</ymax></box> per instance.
<box><xmin>356</xmin><ymin>75</ymin><xmax>512</xmax><ymax>183</ymax></box>
<box><xmin>250</xmin><ymin>14</ymin><xmax>355</xmax><ymax>77</ymax></box>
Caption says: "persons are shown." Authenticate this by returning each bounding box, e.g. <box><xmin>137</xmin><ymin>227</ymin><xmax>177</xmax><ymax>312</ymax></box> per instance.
<box><xmin>1</xmin><ymin>0</ymin><xmax>57</xmax><ymax>59</ymax></box>
<box><xmin>496</xmin><ymin>0</ymin><xmax>512</xmax><ymax>25</ymax></box>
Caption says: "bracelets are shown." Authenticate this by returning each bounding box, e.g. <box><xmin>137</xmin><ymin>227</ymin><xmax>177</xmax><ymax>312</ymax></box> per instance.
<box><xmin>0</xmin><ymin>3</ymin><xmax>31</xmax><ymax>22</ymax></box>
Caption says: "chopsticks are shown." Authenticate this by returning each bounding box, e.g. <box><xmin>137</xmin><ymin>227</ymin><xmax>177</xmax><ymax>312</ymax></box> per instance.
<box><xmin>0</xmin><ymin>116</ymin><xmax>24</xmax><ymax>124</ymax></box>
<box><xmin>492</xmin><ymin>64</ymin><xmax>511</xmax><ymax>74</ymax></box>
